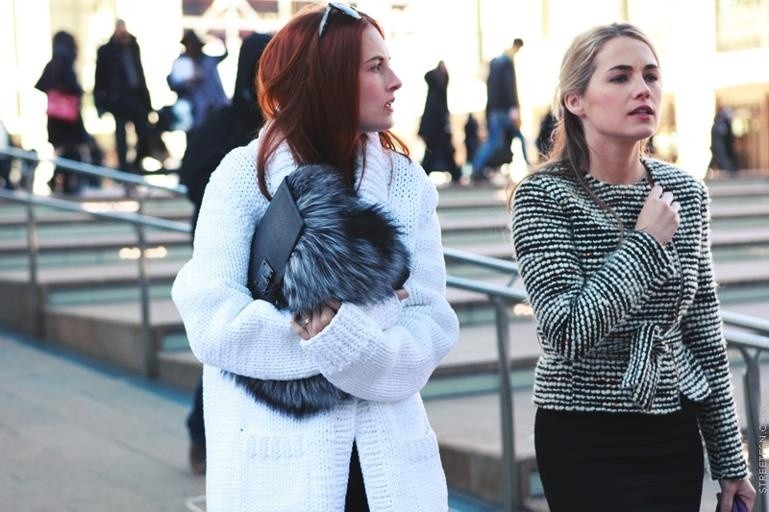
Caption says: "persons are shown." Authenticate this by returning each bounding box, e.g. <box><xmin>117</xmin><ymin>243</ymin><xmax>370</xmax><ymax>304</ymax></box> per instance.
<box><xmin>414</xmin><ymin>35</ymin><xmax>531</xmax><ymax>186</ymax></box>
<box><xmin>34</xmin><ymin>18</ymin><xmax>225</xmax><ymax>195</ymax></box>
<box><xmin>173</xmin><ymin>4</ymin><xmax>466</xmax><ymax>512</ymax></box>
<box><xmin>185</xmin><ymin>31</ymin><xmax>282</xmax><ymax>477</ymax></box>
<box><xmin>509</xmin><ymin>21</ymin><xmax>755</xmax><ymax>512</ymax></box>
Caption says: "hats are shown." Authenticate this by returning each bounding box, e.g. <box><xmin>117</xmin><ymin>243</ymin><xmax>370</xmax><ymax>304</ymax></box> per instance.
<box><xmin>180</xmin><ymin>31</ymin><xmax>205</xmax><ymax>48</ymax></box>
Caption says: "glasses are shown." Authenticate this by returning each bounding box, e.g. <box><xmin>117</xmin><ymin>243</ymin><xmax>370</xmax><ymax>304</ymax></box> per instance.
<box><xmin>317</xmin><ymin>2</ymin><xmax>363</xmax><ymax>37</ymax></box>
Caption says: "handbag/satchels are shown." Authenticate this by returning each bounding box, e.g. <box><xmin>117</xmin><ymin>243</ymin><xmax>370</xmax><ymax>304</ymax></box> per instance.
<box><xmin>486</xmin><ymin>143</ymin><xmax>513</xmax><ymax>166</ymax></box>
<box><xmin>135</xmin><ymin>121</ymin><xmax>169</xmax><ymax>161</ymax></box>
<box><xmin>159</xmin><ymin>106</ymin><xmax>187</xmax><ymax>132</ymax></box>
<box><xmin>227</xmin><ymin>172</ymin><xmax>413</xmax><ymax>416</ymax></box>
